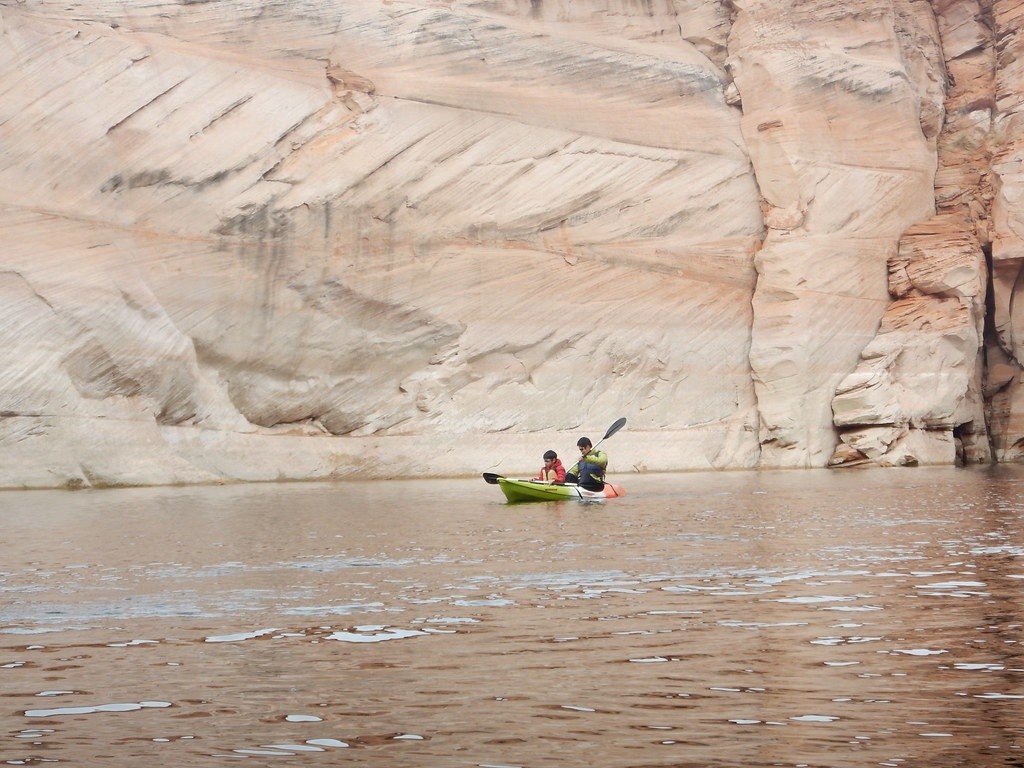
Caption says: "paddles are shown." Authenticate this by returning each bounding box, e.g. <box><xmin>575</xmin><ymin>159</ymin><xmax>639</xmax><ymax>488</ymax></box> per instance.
<box><xmin>482</xmin><ymin>472</ymin><xmax>580</xmax><ymax>487</ymax></box>
<box><xmin>565</xmin><ymin>417</ymin><xmax>626</xmax><ymax>473</ymax></box>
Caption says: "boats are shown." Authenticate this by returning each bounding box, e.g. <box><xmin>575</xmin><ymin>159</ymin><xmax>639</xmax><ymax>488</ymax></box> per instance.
<box><xmin>496</xmin><ymin>477</ymin><xmax>627</xmax><ymax>504</ymax></box>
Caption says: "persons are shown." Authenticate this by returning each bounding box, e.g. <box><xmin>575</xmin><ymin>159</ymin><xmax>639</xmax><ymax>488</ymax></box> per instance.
<box><xmin>567</xmin><ymin>437</ymin><xmax>609</xmax><ymax>492</ymax></box>
<box><xmin>529</xmin><ymin>449</ymin><xmax>566</xmax><ymax>486</ymax></box>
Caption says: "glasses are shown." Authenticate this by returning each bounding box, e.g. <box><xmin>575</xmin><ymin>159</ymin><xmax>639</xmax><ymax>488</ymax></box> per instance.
<box><xmin>579</xmin><ymin>447</ymin><xmax>583</xmax><ymax>450</ymax></box>
<box><xmin>545</xmin><ymin>460</ymin><xmax>549</xmax><ymax>462</ymax></box>
